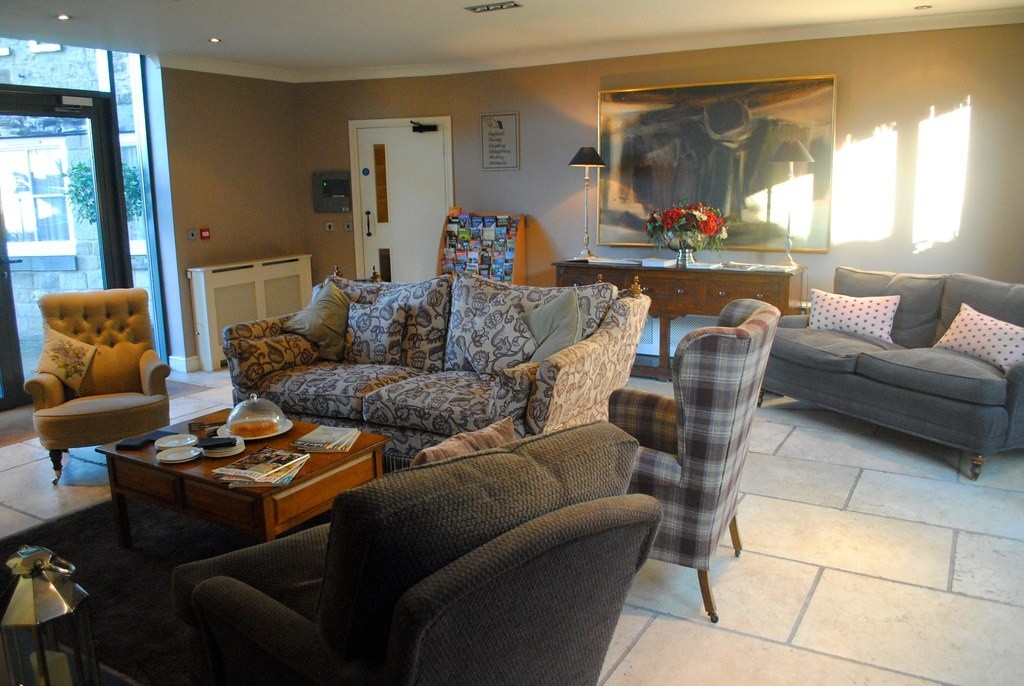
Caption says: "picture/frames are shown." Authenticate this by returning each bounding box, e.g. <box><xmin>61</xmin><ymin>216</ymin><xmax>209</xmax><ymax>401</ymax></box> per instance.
<box><xmin>595</xmin><ymin>73</ymin><xmax>838</xmax><ymax>255</ymax></box>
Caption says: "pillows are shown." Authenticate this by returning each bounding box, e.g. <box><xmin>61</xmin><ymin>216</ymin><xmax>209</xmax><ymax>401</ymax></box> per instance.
<box><xmin>282</xmin><ymin>281</ymin><xmax>408</xmax><ymax>366</ymax></box>
<box><xmin>452</xmin><ymin>285</ymin><xmax>583</xmax><ymax>382</ymax></box>
<box><xmin>34</xmin><ymin>324</ymin><xmax>101</xmax><ymax>396</ymax></box>
<box><xmin>807</xmin><ymin>288</ymin><xmax>901</xmax><ymax>344</ymax></box>
<box><xmin>930</xmin><ymin>302</ymin><xmax>1024</xmax><ymax>374</ymax></box>
<box><xmin>409</xmin><ymin>415</ymin><xmax>516</xmax><ymax>467</ymax></box>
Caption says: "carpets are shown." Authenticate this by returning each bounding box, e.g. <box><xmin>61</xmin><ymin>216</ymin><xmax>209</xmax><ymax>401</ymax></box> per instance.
<box><xmin>0</xmin><ymin>499</ymin><xmax>333</xmax><ymax>685</ymax></box>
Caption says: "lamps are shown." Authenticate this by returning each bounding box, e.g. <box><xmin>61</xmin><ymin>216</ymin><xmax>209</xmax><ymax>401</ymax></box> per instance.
<box><xmin>568</xmin><ymin>145</ymin><xmax>606</xmax><ymax>261</ymax></box>
<box><xmin>769</xmin><ymin>141</ymin><xmax>815</xmax><ymax>264</ymax></box>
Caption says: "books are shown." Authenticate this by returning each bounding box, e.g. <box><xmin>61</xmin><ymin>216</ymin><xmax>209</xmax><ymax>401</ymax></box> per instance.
<box><xmin>211</xmin><ymin>445</ymin><xmax>311</xmax><ymax>487</ymax></box>
<box><xmin>290</xmin><ymin>424</ymin><xmax>362</xmax><ymax>453</ymax></box>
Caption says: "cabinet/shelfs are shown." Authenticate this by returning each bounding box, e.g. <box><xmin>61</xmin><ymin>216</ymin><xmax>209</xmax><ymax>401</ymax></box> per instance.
<box><xmin>436</xmin><ymin>211</ymin><xmax>527</xmax><ymax>285</ymax></box>
<box><xmin>550</xmin><ymin>260</ymin><xmax>806</xmax><ymax>382</ymax></box>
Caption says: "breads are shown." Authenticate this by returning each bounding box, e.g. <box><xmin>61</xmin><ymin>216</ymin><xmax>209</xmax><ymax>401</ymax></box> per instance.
<box><xmin>229</xmin><ymin>421</ymin><xmax>279</xmax><ymax>438</ymax></box>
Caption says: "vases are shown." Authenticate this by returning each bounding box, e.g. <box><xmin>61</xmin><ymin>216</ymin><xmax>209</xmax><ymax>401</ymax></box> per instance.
<box><xmin>666</xmin><ymin>231</ymin><xmax>710</xmax><ymax>264</ymax></box>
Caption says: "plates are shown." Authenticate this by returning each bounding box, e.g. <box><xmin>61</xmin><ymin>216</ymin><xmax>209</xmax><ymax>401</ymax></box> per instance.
<box><xmin>216</xmin><ymin>418</ymin><xmax>293</xmax><ymax>441</ymax></box>
<box><xmin>155</xmin><ymin>447</ymin><xmax>202</xmax><ymax>463</ymax></box>
<box><xmin>155</xmin><ymin>433</ymin><xmax>199</xmax><ymax>450</ymax></box>
<box><xmin>201</xmin><ymin>436</ymin><xmax>246</xmax><ymax>457</ymax></box>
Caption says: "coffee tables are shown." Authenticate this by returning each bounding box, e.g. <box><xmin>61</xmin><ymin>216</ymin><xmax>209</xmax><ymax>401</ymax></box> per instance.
<box><xmin>96</xmin><ymin>408</ymin><xmax>391</xmax><ymax>550</ymax></box>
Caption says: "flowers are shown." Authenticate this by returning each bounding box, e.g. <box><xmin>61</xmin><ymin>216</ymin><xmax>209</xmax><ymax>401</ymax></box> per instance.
<box><xmin>645</xmin><ymin>198</ymin><xmax>729</xmax><ymax>254</ymax></box>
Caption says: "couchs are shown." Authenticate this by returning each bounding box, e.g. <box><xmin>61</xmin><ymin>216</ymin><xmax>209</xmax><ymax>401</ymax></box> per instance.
<box><xmin>169</xmin><ymin>420</ymin><xmax>664</xmax><ymax>686</ymax></box>
<box><xmin>758</xmin><ymin>265</ymin><xmax>1024</xmax><ymax>481</ymax></box>
<box><xmin>222</xmin><ymin>274</ymin><xmax>651</xmax><ymax>475</ymax></box>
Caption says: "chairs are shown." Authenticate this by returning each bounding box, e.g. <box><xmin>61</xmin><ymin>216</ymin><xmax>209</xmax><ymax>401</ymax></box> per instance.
<box><xmin>608</xmin><ymin>298</ymin><xmax>781</xmax><ymax>625</ymax></box>
<box><xmin>23</xmin><ymin>289</ymin><xmax>172</xmax><ymax>485</ymax></box>
